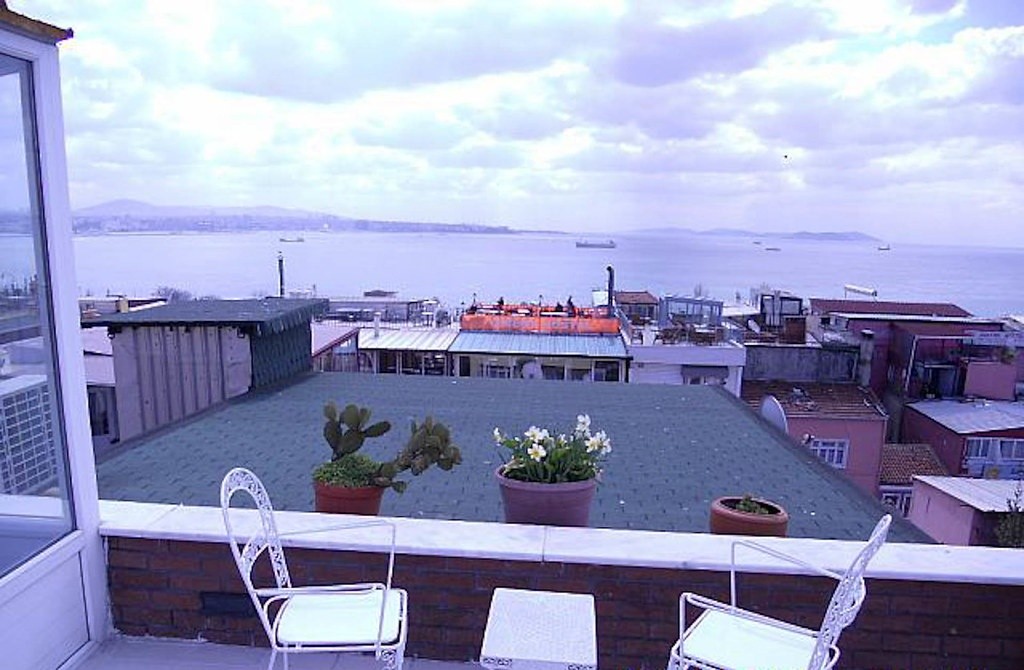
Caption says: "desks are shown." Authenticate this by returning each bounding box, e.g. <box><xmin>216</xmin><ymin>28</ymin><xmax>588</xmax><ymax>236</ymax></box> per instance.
<box><xmin>480</xmin><ymin>588</ymin><xmax>597</xmax><ymax>670</ymax></box>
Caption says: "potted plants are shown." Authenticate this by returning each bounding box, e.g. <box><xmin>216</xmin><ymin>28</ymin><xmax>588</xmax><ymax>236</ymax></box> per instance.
<box><xmin>711</xmin><ymin>494</ymin><xmax>790</xmax><ymax>539</ymax></box>
<box><xmin>311</xmin><ymin>403</ymin><xmax>463</xmax><ymax>515</ymax></box>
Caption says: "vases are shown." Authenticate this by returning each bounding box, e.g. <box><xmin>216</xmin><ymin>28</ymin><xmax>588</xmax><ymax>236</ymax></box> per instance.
<box><xmin>495</xmin><ymin>462</ymin><xmax>601</xmax><ymax>527</ymax></box>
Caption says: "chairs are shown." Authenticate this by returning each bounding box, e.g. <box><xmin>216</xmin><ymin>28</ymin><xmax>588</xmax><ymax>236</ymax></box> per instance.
<box><xmin>665</xmin><ymin>514</ymin><xmax>891</xmax><ymax>670</ymax></box>
<box><xmin>220</xmin><ymin>467</ymin><xmax>408</xmax><ymax>670</ymax></box>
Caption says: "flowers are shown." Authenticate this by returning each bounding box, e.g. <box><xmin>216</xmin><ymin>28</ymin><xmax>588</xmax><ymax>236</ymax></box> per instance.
<box><xmin>491</xmin><ymin>414</ymin><xmax>612</xmax><ymax>482</ymax></box>
